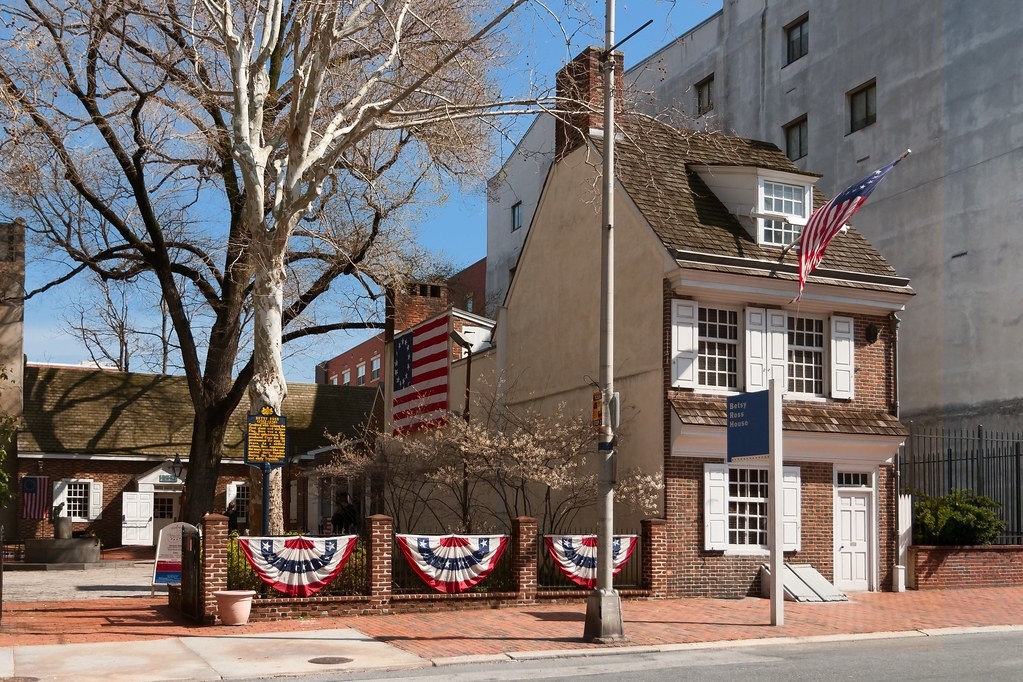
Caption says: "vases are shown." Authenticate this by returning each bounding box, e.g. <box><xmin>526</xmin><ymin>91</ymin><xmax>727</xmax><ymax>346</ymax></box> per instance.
<box><xmin>213</xmin><ymin>589</ymin><xmax>257</xmax><ymax>626</ymax></box>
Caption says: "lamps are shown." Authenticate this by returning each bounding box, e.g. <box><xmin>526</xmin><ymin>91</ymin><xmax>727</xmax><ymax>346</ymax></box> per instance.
<box><xmin>171</xmin><ymin>453</ymin><xmax>184</xmax><ymax>478</ymax></box>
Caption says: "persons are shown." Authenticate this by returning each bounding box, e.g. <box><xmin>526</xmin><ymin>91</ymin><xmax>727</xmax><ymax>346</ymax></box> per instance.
<box><xmin>330</xmin><ymin>499</ymin><xmax>355</xmax><ymax>535</ymax></box>
<box><xmin>224</xmin><ymin>502</ymin><xmax>238</xmax><ymax>538</ymax></box>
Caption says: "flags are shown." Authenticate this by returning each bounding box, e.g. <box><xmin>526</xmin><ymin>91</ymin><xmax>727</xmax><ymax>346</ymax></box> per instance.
<box><xmin>392</xmin><ymin>313</ymin><xmax>449</xmax><ymax>436</ymax></box>
<box><xmin>787</xmin><ymin>155</ymin><xmax>903</xmax><ymax>304</ymax></box>
<box><xmin>22</xmin><ymin>475</ymin><xmax>51</xmax><ymax>519</ymax></box>
